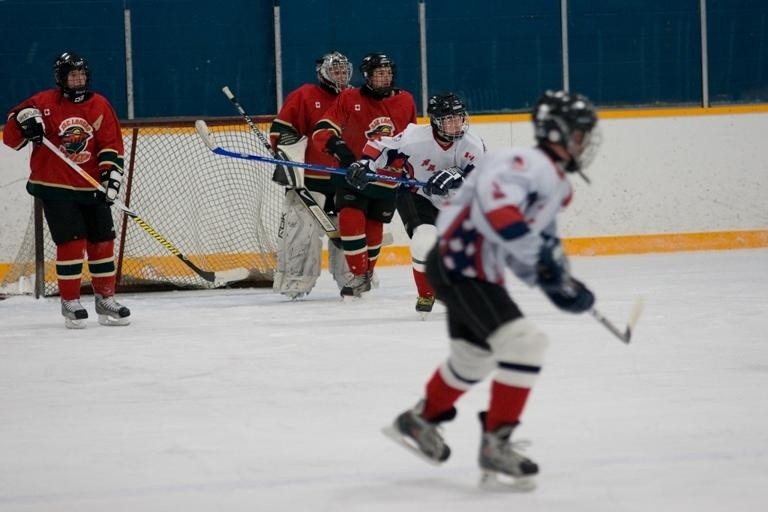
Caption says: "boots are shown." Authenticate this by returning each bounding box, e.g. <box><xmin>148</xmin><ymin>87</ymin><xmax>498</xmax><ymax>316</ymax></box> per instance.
<box><xmin>479</xmin><ymin>411</ymin><xmax>537</xmax><ymax>475</ymax></box>
<box><xmin>396</xmin><ymin>398</ymin><xmax>455</xmax><ymax>461</ymax></box>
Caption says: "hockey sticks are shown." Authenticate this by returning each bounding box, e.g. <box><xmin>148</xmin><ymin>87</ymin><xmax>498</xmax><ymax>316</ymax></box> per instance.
<box><xmin>194</xmin><ymin>117</ymin><xmax>431</xmax><ymax>186</ymax></box>
<box><xmin>42</xmin><ymin>135</ymin><xmax>249</xmax><ymax>281</ymax></box>
<box><xmin>222</xmin><ymin>86</ymin><xmax>393</xmax><ymax>251</ymax></box>
<box><xmin>586</xmin><ymin>295</ymin><xmax>644</xmax><ymax>343</ymax></box>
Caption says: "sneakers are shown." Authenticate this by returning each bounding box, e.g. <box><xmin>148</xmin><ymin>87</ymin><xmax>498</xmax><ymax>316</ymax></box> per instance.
<box><xmin>341</xmin><ymin>273</ymin><xmax>371</xmax><ymax>296</ymax></box>
<box><xmin>61</xmin><ymin>296</ymin><xmax>88</xmax><ymax>320</ymax></box>
<box><xmin>94</xmin><ymin>293</ymin><xmax>130</xmax><ymax>317</ymax></box>
<box><xmin>416</xmin><ymin>295</ymin><xmax>435</xmax><ymax>312</ymax></box>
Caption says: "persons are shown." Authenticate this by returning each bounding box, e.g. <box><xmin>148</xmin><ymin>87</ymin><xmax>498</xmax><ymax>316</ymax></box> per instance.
<box><xmin>4</xmin><ymin>51</ymin><xmax>130</xmax><ymax>320</ymax></box>
<box><xmin>345</xmin><ymin>91</ymin><xmax>488</xmax><ymax>313</ymax></box>
<box><xmin>269</xmin><ymin>51</ymin><xmax>362</xmax><ymax>299</ymax></box>
<box><xmin>391</xmin><ymin>88</ymin><xmax>597</xmax><ymax>477</ymax></box>
<box><xmin>312</xmin><ymin>52</ymin><xmax>418</xmax><ymax>298</ymax></box>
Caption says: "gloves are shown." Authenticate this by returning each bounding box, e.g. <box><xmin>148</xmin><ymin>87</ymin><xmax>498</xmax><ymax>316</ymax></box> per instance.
<box><xmin>346</xmin><ymin>158</ymin><xmax>374</xmax><ymax>190</ymax></box>
<box><xmin>325</xmin><ymin>137</ymin><xmax>356</xmax><ymax>167</ymax></box>
<box><xmin>427</xmin><ymin>166</ymin><xmax>465</xmax><ymax>195</ymax></box>
<box><xmin>94</xmin><ymin>163</ymin><xmax>121</xmax><ymax>206</ymax></box>
<box><xmin>15</xmin><ymin>107</ymin><xmax>47</xmax><ymax>144</ymax></box>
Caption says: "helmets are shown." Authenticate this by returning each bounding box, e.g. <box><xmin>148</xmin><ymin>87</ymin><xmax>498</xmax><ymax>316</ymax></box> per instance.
<box><xmin>428</xmin><ymin>93</ymin><xmax>469</xmax><ymax>142</ymax></box>
<box><xmin>55</xmin><ymin>53</ymin><xmax>89</xmax><ymax>103</ymax></box>
<box><xmin>533</xmin><ymin>90</ymin><xmax>599</xmax><ymax>170</ymax></box>
<box><xmin>360</xmin><ymin>54</ymin><xmax>394</xmax><ymax>97</ymax></box>
<box><xmin>317</xmin><ymin>51</ymin><xmax>353</xmax><ymax>94</ymax></box>
